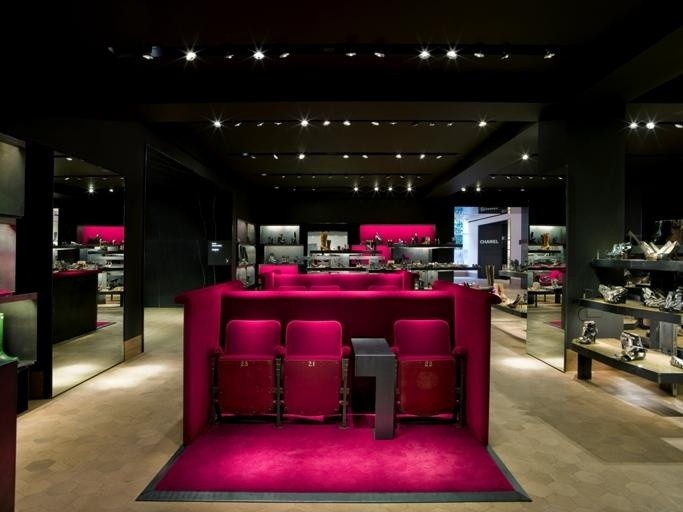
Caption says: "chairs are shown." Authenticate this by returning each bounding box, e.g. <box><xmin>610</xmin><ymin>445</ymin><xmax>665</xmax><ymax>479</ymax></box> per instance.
<box><xmin>173</xmin><ymin>265</ymin><xmax>494</xmax><ymax>445</ymax></box>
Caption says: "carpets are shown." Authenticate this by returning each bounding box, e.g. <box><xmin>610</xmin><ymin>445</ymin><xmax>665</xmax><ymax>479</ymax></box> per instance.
<box><xmin>136</xmin><ymin>416</ymin><xmax>532</xmax><ymax>504</ymax></box>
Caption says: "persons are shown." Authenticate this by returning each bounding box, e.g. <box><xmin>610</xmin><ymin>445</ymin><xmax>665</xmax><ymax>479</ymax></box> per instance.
<box><xmin>652</xmin><ymin>220</ymin><xmax>682</xmax><ymax>296</ymax></box>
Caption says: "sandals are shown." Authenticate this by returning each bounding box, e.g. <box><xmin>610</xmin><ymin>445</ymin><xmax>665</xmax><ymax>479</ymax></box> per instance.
<box><xmin>597</xmin><ymin>268</ymin><xmax>682</xmax><ymax>312</ymax></box>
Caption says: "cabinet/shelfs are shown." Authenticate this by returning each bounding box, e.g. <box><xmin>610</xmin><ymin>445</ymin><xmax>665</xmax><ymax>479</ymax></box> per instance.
<box><xmin>490</xmin><ymin>239</ymin><xmax>526</xmax><ymax>321</ymax></box>
<box><xmin>0</xmin><ymin>134</ymin><xmax>40</xmax><ymax>414</ymax></box>
<box><xmin>52</xmin><ymin>235</ymin><xmax>124</xmax><ymax>307</ymax></box>
<box><xmin>567</xmin><ymin>256</ymin><xmax>682</xmax><ymax>392</ymax></box>
<box><xmin>232</xmin><ymin>215</ymin><xmax>481</xmax><ymax>288</ymax></box>
<box><xmin>529</xmin><ymin>226</ymin><xmax>563</xmax><ymax>310</ymax></box>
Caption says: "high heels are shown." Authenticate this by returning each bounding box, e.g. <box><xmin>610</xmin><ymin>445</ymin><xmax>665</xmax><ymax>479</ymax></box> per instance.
<box><xmin>576</xmin><ymin>320</ymin><xmax>645</xmax><ymax>361</ymax></box>
<box><xmin>604</xmin><ymin>240</ymin><xmax>680</xmax><ymax>261</ymax></box>
<box><xmin>508</xmin><ymin>295</ymin><xmax>522</xmax><ymax>308</ymax></box>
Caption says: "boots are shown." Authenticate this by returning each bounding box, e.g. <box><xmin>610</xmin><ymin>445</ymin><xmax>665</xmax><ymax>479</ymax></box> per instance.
<box><xmin>319</xmin><ymin>232</ymin><xmax>329</xmax><ymax>250</ymax></box>
<box><xmin>538</xmin><ymin>234</ymin><xmax>549</xmax><ymax>250</ymax></box>
<box><xmin>267</xmin><ymin>232</ymin><xmax>296</xmax><ymax>245</ymax></box>
<box><xmin>243</xmin><ymin>247</ymin><xmax>248</xmax><ymax>262</ymax></box>
<box><xmin>326</xmin><ymin>240</ymin><xmax>330</xmax><ymax>248</ymax></box>
<box><xmin>485</xmin><ymin>265</ymin><xmax>507</xmax><ymax>302</ymax></box>
<box><xmin>240</xmin><ymin>245</ymin><xmax>244</xmax><ymax>258</ymax></box>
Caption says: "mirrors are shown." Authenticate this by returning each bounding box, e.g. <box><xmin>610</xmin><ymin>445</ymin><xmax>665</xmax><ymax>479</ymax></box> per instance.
<box><xmin>525</xmin><ymin>164</ymin><xmax>568</xmax><ymax>374</ymax></box>
<box><xmin>49</xmin><ymin>149</ymin><xmax>128</xmax><ymax>400</ymax></box>
